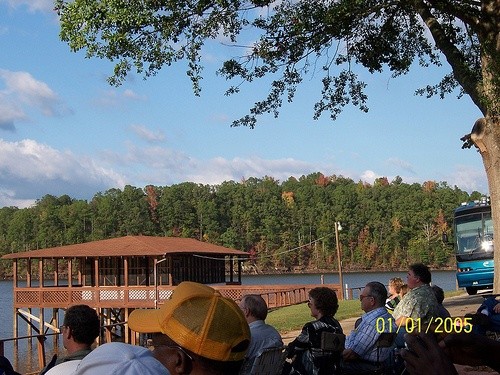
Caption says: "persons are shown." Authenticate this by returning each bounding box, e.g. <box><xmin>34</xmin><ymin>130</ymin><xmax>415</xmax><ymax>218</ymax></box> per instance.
<box><xmin>355</xmin><ymin>263</ymin><xmax>500</xmax><ymax>375</ymax></box>
<box><xmin>342</xmin><ymin>281</ymin><xmax>398</xmax><ymax>375</ymax></box>
<box><xmin>282</xmin><ymin>287</ymin><xmax>344</xmax><ymax>375</ymax></box>
<box><xmin>39</xmin><ymin>304</ymin><xmax>100</xmax><ymax>375</ymax></box>
<box><xmin>0</xmin><ymin>356</ymin><xmax>22</xmax><ymax>375</ymax></box>
<box><xmin>45</xmin><ymin>342</ymin><xmax>171</xmax><ymax>375</ymax></box>
<box><xmin>237</xmin><ymin>293</ymin><xmax>283</xmax><ymax>375</ymax></box>
<box><xmin>127</xmin><ymin>282</ymin><xmax>252</xmax><ymax>375</ymax></box>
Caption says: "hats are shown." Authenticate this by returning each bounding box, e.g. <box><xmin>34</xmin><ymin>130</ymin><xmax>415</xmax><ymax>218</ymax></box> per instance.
<box><xmin>128</xmin><ymin>281</ymin><xmax>251</xmax><ymax>360</ymax></box>
<box><xmin>44</xmin><ymin>342</ymin><xmax>171</xmax><ymax>375</ymax></box>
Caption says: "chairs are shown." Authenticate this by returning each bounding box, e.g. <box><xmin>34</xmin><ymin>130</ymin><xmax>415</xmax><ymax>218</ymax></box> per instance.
<box><xmin>247</xmin><ymin>332</ymin><xmax>397</xmax><ymax>375</ymax></box>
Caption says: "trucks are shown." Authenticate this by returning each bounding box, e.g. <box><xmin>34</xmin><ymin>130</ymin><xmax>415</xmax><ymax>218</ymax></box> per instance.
<box><xmin>442</xmin><ymin>196</ymin><xmax>495</xmax><ymax>296</ymax></box>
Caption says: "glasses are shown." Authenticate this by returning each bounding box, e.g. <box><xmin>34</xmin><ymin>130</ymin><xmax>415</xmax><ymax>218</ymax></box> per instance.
<box><xmin>360</xmin><ymin>295</ymin><xmax>370</xmax><ymax>301</ymax></box>
<box><xmin>146</xmin><ymin>339</ymin><xmax>194</xmax><ymax>360</ymax></box>
<box><xmin>307</xmin><ymin>302</ymin><xmax>314</xmax><ymax>308</ymax></box>
<box><xmin>59</xmin><ymin>326</ymin><xmax>68</xmax><ymax>332</ymax></box>
